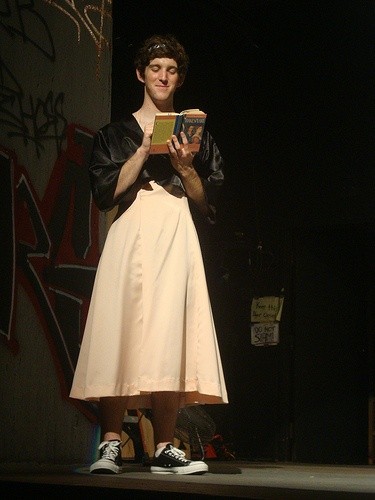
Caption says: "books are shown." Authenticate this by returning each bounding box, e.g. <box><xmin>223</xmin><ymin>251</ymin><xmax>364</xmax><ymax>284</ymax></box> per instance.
<box><xmin>149</xmin><ymin>108</ymin><xmax>207</xmax><ymax>154</ymax></box>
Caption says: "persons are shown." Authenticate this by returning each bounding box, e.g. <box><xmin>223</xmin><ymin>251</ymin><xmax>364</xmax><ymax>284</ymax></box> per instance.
<box><xmin>71</xmin><ymin>37</ymin><xmax>228</xmax><ymax>475</ymax></box>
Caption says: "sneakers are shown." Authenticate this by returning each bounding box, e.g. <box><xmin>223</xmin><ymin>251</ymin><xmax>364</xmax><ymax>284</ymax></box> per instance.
<box><xmin>150</xmin><ymin>443</ymin><xmax>208</xmax><ymax>475</ymax></box>
<box><xmin>90</xmin><ymin>441</ymin><xmax>124</xmax><ymax>474</ymax></box>
